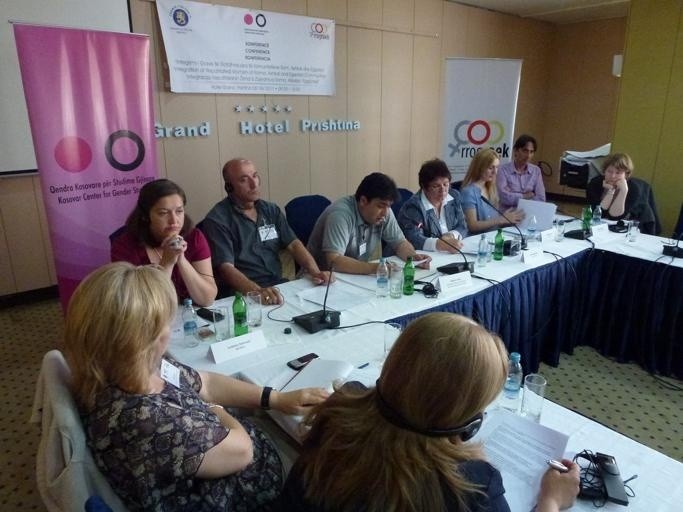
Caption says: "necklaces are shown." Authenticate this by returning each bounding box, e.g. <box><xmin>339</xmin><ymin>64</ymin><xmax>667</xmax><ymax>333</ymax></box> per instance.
<box><xmin>154</xmin><ymin>248</ymin><xmax>164</xmax><ymax>260</ymax></box>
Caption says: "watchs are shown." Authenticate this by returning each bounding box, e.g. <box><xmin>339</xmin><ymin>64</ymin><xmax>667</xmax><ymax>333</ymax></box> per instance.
<box><xmin>260</xmin><ymin>386</ymin><xmax>276</xmax><ymax>410</ymax></box>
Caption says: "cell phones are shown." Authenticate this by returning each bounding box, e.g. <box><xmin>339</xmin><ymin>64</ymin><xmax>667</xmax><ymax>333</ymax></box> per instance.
<box><xmin>287</xmin><ymin>353</ymin><xmax>320</xmax><ymax>371</ymax></box>
<box><xmin>196</xmin><ymin>307</ymin><xmax>224</xmax><ymax>323</ymax></box>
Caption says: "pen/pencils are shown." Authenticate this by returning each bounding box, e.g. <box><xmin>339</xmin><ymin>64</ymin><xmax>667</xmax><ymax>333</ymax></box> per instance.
<box><xmin>546</xmin><ymin>458</ymin><xmax>570</xmax><ymax>472</ymax></box>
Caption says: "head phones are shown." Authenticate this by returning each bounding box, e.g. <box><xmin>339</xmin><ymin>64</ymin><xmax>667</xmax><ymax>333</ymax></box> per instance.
<box><xmin>375</xmin><ymin>378</ymin><xmax>483</xmax><ymax>444</ymax></box>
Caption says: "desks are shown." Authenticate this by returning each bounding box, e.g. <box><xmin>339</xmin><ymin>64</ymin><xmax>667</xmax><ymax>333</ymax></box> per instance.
<box><xmin>161</xmin><ymin>211</ymin><xmax>682</xmax><ymax>512</ymax></box>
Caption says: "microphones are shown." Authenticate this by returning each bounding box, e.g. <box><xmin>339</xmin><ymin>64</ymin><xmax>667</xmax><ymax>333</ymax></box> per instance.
<box><xmin>481</xmin><ymin>195</ymin><xmax>527</xmax><ymax>254</ymax></box>
<box><xmin>556</xmin><ymin>209</ymin><xmax>594</xmax><ymax>239</ymax></box>
<box><xmin>411</xmin><ymin>220</ymin><xmax>475</xmax><ymax>274</ymax></box>
<box><xmin>293</xmin><ymin>260</ymin><xmax>342</xmax><ymax>334</ymax></box>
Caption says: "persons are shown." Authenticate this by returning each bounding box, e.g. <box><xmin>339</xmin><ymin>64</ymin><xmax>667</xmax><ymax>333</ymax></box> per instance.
<box><xmin>383</xmin><ymin>158</ymin><xmax>469</xmax><ymax>254</ymax></box>
<box><xmin>274</xmin><ymin>310</ymin><xmax>581</xmax><ymax>512</ymax></box>
<box><xmin>110</xmin><ymin>180</ymin><xmax>217</xmax><ymax>306</ymax></box>
<box><xmin>497</xmin><ymin>135</ymin><xmax>545</xmax><ymax>212</ymax></box>
<box><xmin>202</xmin><ymin>158</ymin><xmax>335</xmax><ymax>305</ymax></box>
<box><xmin>459</xmin><ymin>148</ymin><xmax>526</xmax><ymax>239</ymax></box>
<box><xmin>586</xmin><ymin>153</ymin><xmax>634</xmax><ymax>220</ymax></box>
<box><xmin>294</xmin><ymin>171</ymin><xmax>432</xmax><ymax>278</ymax></box>
<box><xmin>64</xmin><ymin>260</ymin><xmax>331</xmax><ymax>512</ymax></box>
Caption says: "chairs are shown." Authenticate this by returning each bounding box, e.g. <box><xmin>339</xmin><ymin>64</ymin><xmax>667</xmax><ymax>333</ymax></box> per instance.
<box><xmin>31</xmin><ymin>350</ymin><xmax>124</xmax><ymax>512</ymax></box>
<box><xmin>283</xmin><ymin>195</ymin><xmax>333</xmax><ymax>249</ymax></box>
<box><xmin>389</xmin><ymin>187</ymin><xmax>414</xmax><ymax>220</ymax></box>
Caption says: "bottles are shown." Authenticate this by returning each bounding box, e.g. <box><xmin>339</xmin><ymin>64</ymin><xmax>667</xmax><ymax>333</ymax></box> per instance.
<box><xmin>403</xmin><ymin>256</ymin><xmax>415</xmax><ymax>296</ymax></box>
<box><xmin>182</xmin><ymin>298</ymin><xmax>200</xmax><ymax>348</ymax></box>
<box><xmin>529</xmin><ymin>214</ymin><xmax>537</xmax><ymax>240</ymax></box>
<box><xmin>502</xmin><ymin>352</ymin><xmax>522</xmax><ymax>412</ymax></box>
<box><xmin>494</xmin><ymin>228</ymin><xmax>503</xmax><ymax>260</ymax></box>
<box><xmin>593</xmin><ymin>205</ymin><xmax>602</xmax><ymax>225</ymax></box>
<box><xmin>376</xmin><ymin>257</ymin><xmax>387</xmax><ymax>298</ymax></box>
<box><xmin>232</xmin><ymin>290</ymin><xmax>250</xmax><ymax>336</ymax></box>
<box><xmin>582</xmin><ymin>204</ymin><xmax>593</xmax><ymax>230</ymax></box>
<box><xmin>478</xmin><ymin>233</ymin><xmax>486</xmax><ymax>267</ymax></box>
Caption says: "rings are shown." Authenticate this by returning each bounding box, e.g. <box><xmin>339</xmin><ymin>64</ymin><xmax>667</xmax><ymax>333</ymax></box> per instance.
<box><xmin>265</xmin><ymin>295</ymin><xmax>269</xmax><ymax>300</ymax></box>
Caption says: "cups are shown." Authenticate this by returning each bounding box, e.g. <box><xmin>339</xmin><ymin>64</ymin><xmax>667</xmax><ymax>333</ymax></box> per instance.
<box><xmin>213</xmin><ymin>306</ymin><xmax>231</xmax><ymax>341</ymax></box>
<box><xmin>554</xmin><ymin>217</ymin><xmax>565</xmax><ymax>242</ymax></box>
<box><xmin>520</xmin><ymin>375</ymin><xmax>546</xmax><ymax>424</ymax></box>
<box><xmin>247</xmin><ymin>291</ymin><xmax>262</xmax><ymax>326</ymax></box>
<box><xmin>627</xmin><ymin>221</ymin><xmax>640</xmax><ymax>242</ymax></box>
<box><xmin>389</xmin><ymin>266</ymin><xmax>402</xmax><ymax>299</ymax></box>
